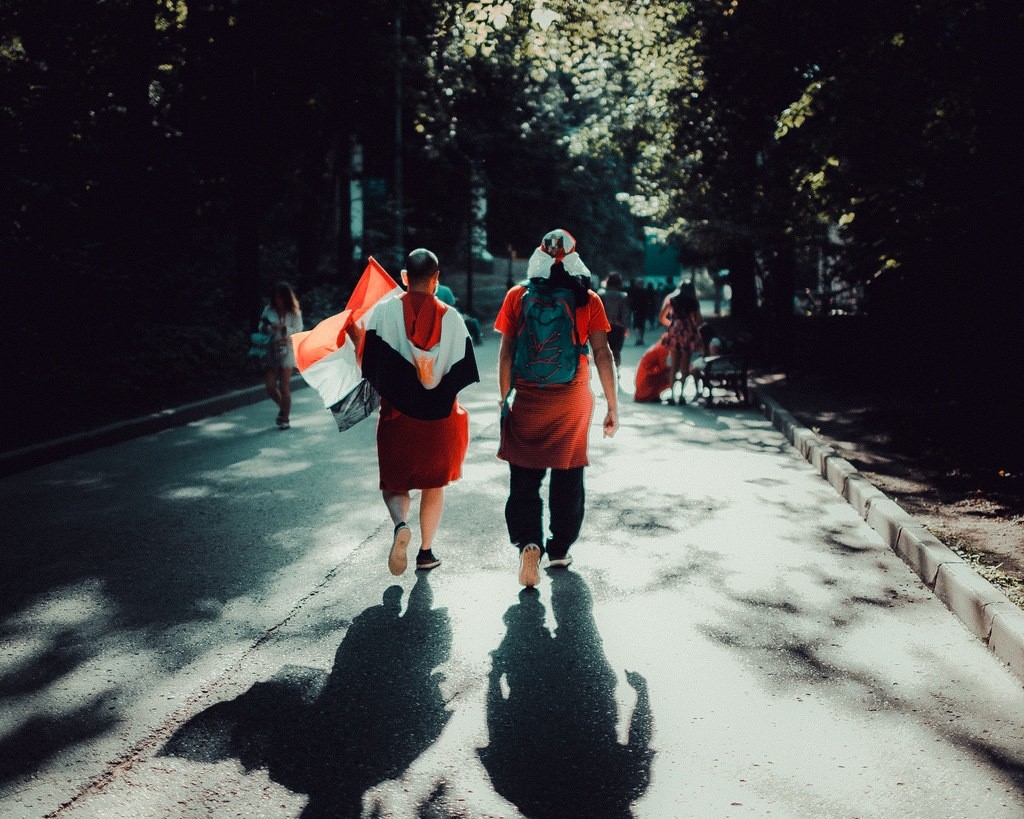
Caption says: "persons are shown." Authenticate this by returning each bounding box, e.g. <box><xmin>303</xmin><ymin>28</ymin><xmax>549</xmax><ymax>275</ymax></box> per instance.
<box><xmin>429</xmin><ymin>272</ymin><xmax>724</xmax><ymax>406</ymax></box>
<box><xmin>361</xmin><ymin>247</ymin><xmax>480</xmax><ymax>576</ymax></box>
<box><xmin>493</xmin><ymin>228</ymin><xmax>620</xmax><ymax>588</ymax></box>
<box><xmin>258</xmin><ymin>281</ymin><xmax>303</xmax><ymax>431</ymax></box>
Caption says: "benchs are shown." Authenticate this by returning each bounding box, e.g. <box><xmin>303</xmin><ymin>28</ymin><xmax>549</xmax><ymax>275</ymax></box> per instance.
<box><xmin>697</xmin><ymin>343</ymin><xmax>749</xmax><ymax>408</ymax></box>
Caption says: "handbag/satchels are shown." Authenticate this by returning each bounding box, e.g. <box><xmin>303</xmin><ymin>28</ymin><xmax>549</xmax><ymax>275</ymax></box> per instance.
<box><xmin>250</xmin><ymin>326</ymin><xmax>271</xmax><ymax>356</ymax></box>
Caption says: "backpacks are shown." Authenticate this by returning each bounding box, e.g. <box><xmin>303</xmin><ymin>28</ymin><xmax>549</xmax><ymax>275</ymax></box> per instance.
<box><xmin>510</xmin><ymin>279</ymin><xmax>589</xmax><ymax>384</ymax></box>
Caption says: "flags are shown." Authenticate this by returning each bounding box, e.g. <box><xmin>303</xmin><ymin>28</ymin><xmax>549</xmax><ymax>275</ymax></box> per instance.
<box><xmin>290</xmin><ymin>259</ymin><xmax>406</xmax><ymax>434</ymax></box>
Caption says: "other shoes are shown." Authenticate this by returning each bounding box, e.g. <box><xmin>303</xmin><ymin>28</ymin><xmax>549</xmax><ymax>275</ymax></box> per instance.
<box><xmin>416</xmin><ymin>553</ymin><xmax>441</xmax><ymax>569</ymax></box>
<box><xmin>518</xmin><ymin>544</ymin><xmax>541</xmax><ymax>586</ymax></box>
<box><xmin>281</xmin><ymin>421</ymin><xmax>290</xmax><ymax>429</ymax></box>
<box><xmin>389</xmin><ymin>527</ymin><xmax>411</xmax><ymax>576</ymax></box>
<box><xmin>548</xmin><ymin>551</ymin><xmax>572</xmax><ymax>567</ymax></box>
<box><xmin>276</xmin><ymin>411</ymin><xmax>283</xmax><ymax>425</ymax></box>
